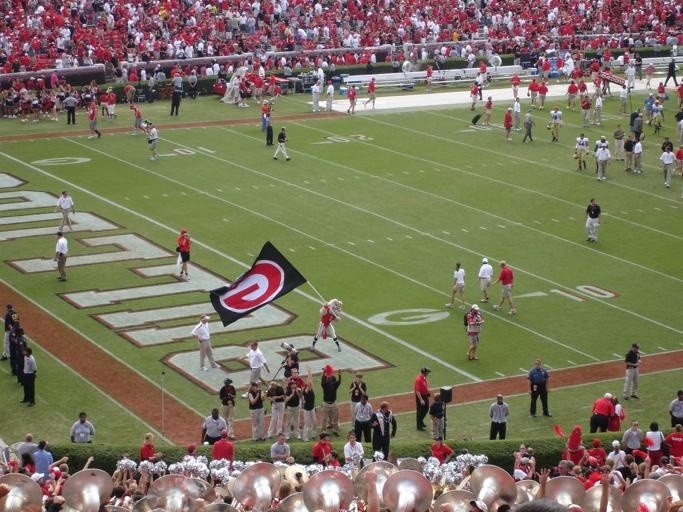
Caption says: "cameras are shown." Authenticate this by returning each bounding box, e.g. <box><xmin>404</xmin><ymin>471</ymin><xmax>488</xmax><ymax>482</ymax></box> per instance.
<box><xmin>145</xmin><ymin>119</ymin><xmax>152</xmax><ymax>125</ymax></box>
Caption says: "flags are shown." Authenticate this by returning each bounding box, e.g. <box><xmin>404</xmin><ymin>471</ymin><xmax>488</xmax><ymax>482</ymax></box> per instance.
<box><xmin>207</xmin><ymin>241</ymin><xmax>307</xmax><ymax>327</ymax></box>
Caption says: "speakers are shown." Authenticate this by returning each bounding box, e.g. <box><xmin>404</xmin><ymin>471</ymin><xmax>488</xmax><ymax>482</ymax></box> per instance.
<box><xmin>440</xmin><ymin>385</ymin><xmax>452</xmax><ymax>403</ymax></box>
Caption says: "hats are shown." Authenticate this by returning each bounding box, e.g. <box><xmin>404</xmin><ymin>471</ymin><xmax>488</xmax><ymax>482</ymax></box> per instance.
<box><xmin>473</xmin><ymin>304</ymin><xmax>480</xmax><ymax>311</ymax></box>
<box><xmin>482</xmin><ymin>257</ymin><xmax>490</xmax><ymax>263</ymax></box>
<box><xmin>521</xmin><ymin>344</ymin><xmax>683</xmax><ymax>474</ymax></box>
<box><xmin>188</xmin><ymin>315</ymin><xmax>442</xmax><ymax>455</ymax></box>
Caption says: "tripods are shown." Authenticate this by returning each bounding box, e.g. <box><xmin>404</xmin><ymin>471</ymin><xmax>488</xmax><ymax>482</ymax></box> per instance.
<box><xmin>268</xmin><ymin>356</ymin><xmax>299</xmax><ymax>387</ymax></box>
<box><xmin>144</xmin><ymin>126</ymin><xmax>151</xmax><ymax>144</ymax></box>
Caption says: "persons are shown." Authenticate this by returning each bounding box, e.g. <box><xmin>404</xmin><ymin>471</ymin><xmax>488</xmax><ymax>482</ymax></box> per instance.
<box><xmin>584</xmin><ymin>199</ymin><xmax>601</xmax><ymax>242</ymax></box>
<box><xmin>444</xmin><ymin>263</ymin><xmax>466</xmax><ymax>309</ymax></box>
<box><xmin>55</xmin><ymin>192</ymin><xmax>76</xmax><ymax>235</ymax></box>
<box><xmin>1</xmin><ymin>298</ymin><xmax>681</xmax><ymax>511</ymax></box>
<box><xmin>54</xmin><ymin>231</ymin><xmax>68</xmax><ymax>281</ymax></box>
<box><xmin>476</xmin><ymin>258</ymin><xmax>494</xmax><ymax>302</ymax></box>
<box><xmin>176</xmin><ymin>230</ymin><xmax>191</xmax><ymax>280</ymax></box>
<box><xmin>491</xmin><ymin>261</ymin><xmax>518</xmax><ymax>314</ymax></box>
<box><xmin>1</xmin><ymin>1</ymin><xmax>683</xmax><ymax>190</ymax></box>
